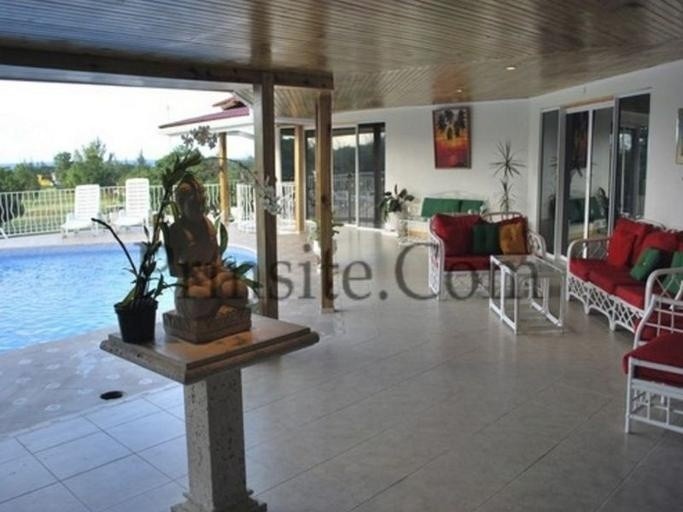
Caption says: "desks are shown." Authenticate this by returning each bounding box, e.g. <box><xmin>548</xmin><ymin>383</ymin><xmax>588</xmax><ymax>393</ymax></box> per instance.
<box><xmin>100</xmin><ymin>313</ymin><xmax>318</xmax><ymax>512</ymax></box>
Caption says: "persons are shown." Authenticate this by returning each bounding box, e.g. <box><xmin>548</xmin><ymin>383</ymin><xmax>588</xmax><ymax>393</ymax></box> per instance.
<box><xmin>158</xmin><ymin>171</ymin><xmax>250</xmax><ymax>318</ymax></box>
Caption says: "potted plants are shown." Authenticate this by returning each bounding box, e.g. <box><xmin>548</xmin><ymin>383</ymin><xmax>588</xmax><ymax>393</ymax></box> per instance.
<box><xmin>307</xmin><ymin>211</ymin><xmax>344</xmax><ymax>260</ymax></box>
<box><xmin>375</xmin><ymin>184</ymin><xmax>414</xmax><ymax>236</ymax></box>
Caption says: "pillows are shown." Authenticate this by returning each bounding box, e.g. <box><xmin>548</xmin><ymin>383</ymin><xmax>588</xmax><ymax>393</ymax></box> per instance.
<box><xmin>676</xmin><ymin>234</ymin><xmax>683</xmax><ymax>252</ymax></box>
<box><xmin>629</xmin><ymin>245</ymin><xmax>663</xmax><ymax>285</ymax></box>
<box><xmin>605</xmin><ymin>231</ymin><xmax>638</xmax><ymax>267</ymax></box>
<box><xmin>434</xmin><ymin>214</ymin><xmax>481</xmax><ymax>257</ymax></box>
<box><xmin>634</xmin><ymin>230</ymin><xmax>675</xmax><ymax>283</ymax></box>
<box><xmin>469</xmin><ymin>222</ymin><xmax>500</xmax><ymax>256</ymax></box>
<box><xmin>497</xmin><ymin>222</ymin><xmax>528</xmax><ymax>254</ymax></box>
<box><xmin>606</xmin><ymin>217</ymin><xmax>652</xmax><ymax>268</ymax></box>
<box><xmin>662</xmin><ymin>251</ymin><xmax>683</xmax><ymax>296</ymax></box>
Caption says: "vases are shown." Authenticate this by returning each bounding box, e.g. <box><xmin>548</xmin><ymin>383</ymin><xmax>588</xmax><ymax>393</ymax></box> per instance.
<box><xmin>114</xmin><ymin>300</ymin><xmax>159</xmax><ymax>344</ymax></box>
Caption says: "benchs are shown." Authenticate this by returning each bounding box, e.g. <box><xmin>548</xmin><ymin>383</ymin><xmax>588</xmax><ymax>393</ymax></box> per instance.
<box><xmin>398</xmin><ymin>190</ymin><xmax>489</xmax><ymax>245</ymax></box>
<box><xmin>427</xmin><ymin>212</ymin><xmax>547</xmax><ymax>301</ymax></box>
<box><xmin>565</xmin><ymin>213</ymin><xmax>683</xmax><ymax>335</ymax></box>
<box><xmin>547</xmin><ymin>190</ymin><xmax>613</xmax><ymax>235</ymax></box>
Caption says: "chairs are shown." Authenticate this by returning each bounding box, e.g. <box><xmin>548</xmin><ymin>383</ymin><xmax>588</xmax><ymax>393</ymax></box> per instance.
<box><xmin>624</xmin><ymin>330</ymin><xmax>683</xmax><ymax>436</ymax></box>
<box><xmin>112</xmin><ymin>178</ymin><xmax>151</xmax><ymax>237</ymax></box>
<box><xmin>633</xmin><ymin>281</ymin><xmax>683</xmax><ymax>351</ymax></box>
<box><xmin>61</xmin><ymin>184</ymin><xmax>100</xmax><ymax>237</ymax></box>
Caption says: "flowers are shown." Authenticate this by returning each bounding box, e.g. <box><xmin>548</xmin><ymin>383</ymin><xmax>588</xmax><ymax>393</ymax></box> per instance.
<box><xmin>92</xmin><ymin>123</ymin><xmax>285</xmax><ymax>301</ymax></box>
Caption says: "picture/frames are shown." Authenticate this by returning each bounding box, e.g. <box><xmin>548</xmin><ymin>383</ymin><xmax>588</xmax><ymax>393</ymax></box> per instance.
<box><xmin>432</xmin><ymin>106</ymin><xmax>471</xmax><ymax>169</ymax></box>
<box><xmin>675</xmin><ymin>106</ymin><xmax>682</xmax><ymax>164</ymax></box>
